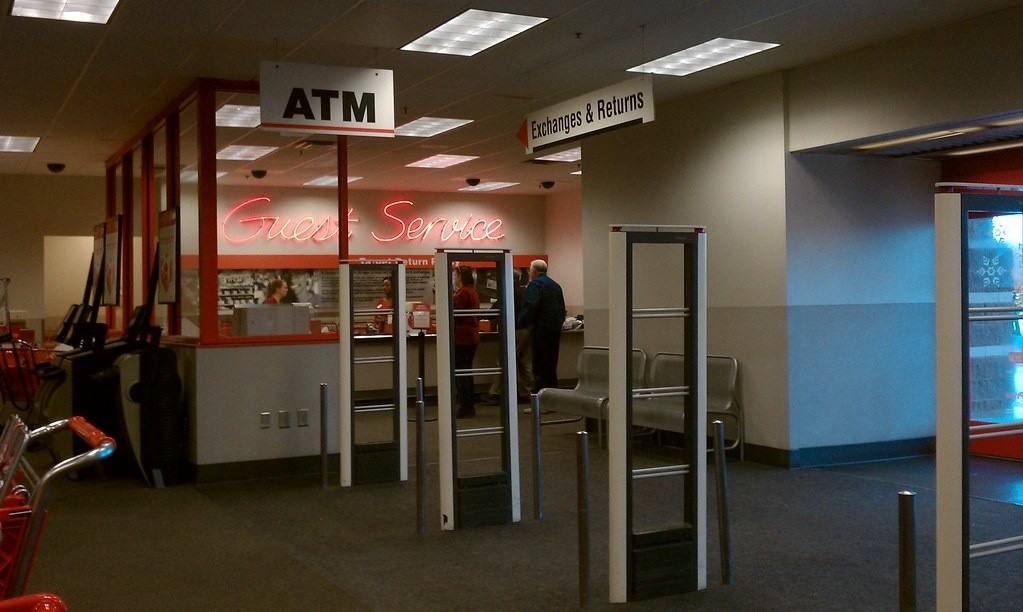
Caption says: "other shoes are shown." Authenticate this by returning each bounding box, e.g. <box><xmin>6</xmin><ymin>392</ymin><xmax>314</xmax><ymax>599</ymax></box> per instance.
<box><xmin>523</xmin><ymin>408</ymin><xmax>548</xmax><ymax>414</ymax></box>
<box><xmin>480</xmin><ymin>394</ymin><xmax>501</xmax><ymax>403</ymax></box>
<box><xmin>456</xmin><ymin>408</ymin><xmax>475</xmax><ymax>418</ymax></box>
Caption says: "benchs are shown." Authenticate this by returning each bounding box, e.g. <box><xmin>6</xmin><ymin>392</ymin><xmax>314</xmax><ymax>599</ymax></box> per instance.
<box><xmin>538</xmin><ymin>345</ymin><xmax>646</xmax><ymax>449</ymax></box>
<box><xmin>606</xmin><ymin>352</ymin><xmax>745</xmax><ymax>468</ymax></box>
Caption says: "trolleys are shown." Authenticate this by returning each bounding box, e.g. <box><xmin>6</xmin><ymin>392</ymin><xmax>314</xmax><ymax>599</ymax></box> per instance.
<box><xmin>0</xmin><ymin>411</ymin><xmax>116</xmax><ymax>602</ymax></box>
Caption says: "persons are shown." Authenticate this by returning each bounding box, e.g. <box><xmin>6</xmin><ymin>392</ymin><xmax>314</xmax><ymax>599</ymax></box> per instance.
<box><xmin>373</xmin><ymin>277</ymin><xmax>394</xmax><ymax>324</ymax></box>
<box><xmin>261</xmin><ymin>280</ymin><xmax>288</xmax><ymax>305</ymax></box>
<box><xmin>517</xmin><ymin>260</ymin><xmax>566</xmax><ymax>414</ymax></box>
<box><xmin>481</xmin><ymin>269</ymin><xmax>534</xmax><ymax>404</ymax></box>
<box><xmin>452</xmin><ymin>266</ymin><xmax>480</xmax><ymax>419</ymax></box>
